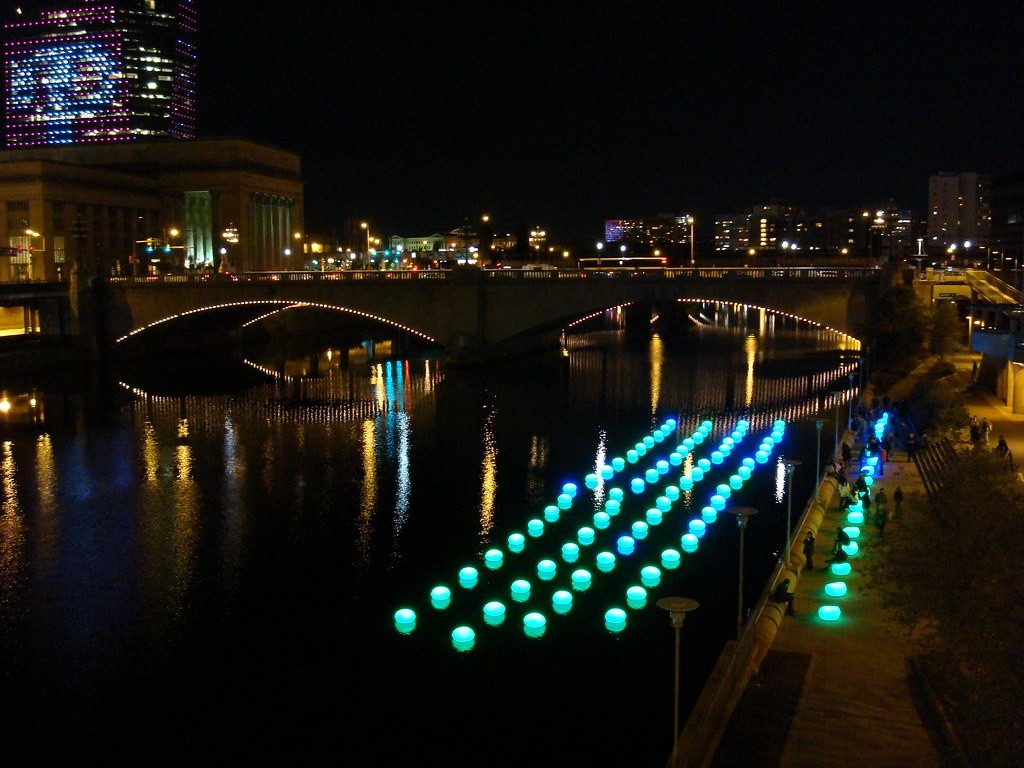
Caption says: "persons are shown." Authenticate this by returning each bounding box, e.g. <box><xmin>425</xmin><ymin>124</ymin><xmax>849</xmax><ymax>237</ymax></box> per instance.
<box><xmin>981</xmin><ymin>417</ymin><xmax>992</xmax><ymax>446</ymax></box>
<box><xmin>822</xmin><ymin>544</ymin><xmax>847</xmax><ymax>572</ymax></box>
<box><xmin>893</xmin><ymin>487</ymin><xmax>904</xmax><ymax>519</ymax></box>
<box><xmin>826</xmin><ymin>466</ymin><xmax>872</xmax><ymax>517</ymax></box>
<box><xmin>775</xmin><ymin>578</ymin><xmax>797</xmax><ymax>616</ymax></box>
<box><xmin>831</xmin><ymin>527</ymin><xmax>850</xmax><ymax>555</ymax></box>
<box><xmin>802</xmin><ymin>531</ymin><xmax>815</xmax><ymax>571</ymax></box>
<box><xmin>872</xmin><ymin>482</ymin><xmax>889</xmax><ymax>536</ymax></box>
<box><xmin>970</xmin><ymin>416</ymin><xmax>980</xmax><ymax>441</ymax></box>
<box><xmin>907</xmin><ymin>437</ymin><xmax>916</xmax><ymax>462</ymax></box>
<box><xmin>996</xmin><ymin>435</ymin><xmax>1008</xmax><ymax>456</ymax></box>
<box><xmin>972</xmin><ymin>361</ymin><xmax>977</xmax><ymax>373</ymax></box>
<box><xmin>841</xmin><ymin>394</ymin><xmax>907</xmax><ymax>476</ymax></box>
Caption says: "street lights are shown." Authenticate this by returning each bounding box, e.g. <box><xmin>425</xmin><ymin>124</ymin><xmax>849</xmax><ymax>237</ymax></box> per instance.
<box><xmin>846</xmin><ymin>373</ymin><xmax>857</xmax><ymax>432</ymax></box>
<box><xmin>727</xmin><ymin>506</ymin><xmax>760</xmax><ymax>640</ymax></box>
<box><xmin>809</xmin><ymin>417</ymin><xmax>830</xmax><ymax>503</ymax></box>
<box><xmin>829</xmin><ymin>389</ymin><xmax>843</xmax><ymax>459</ymax></box>
<box><xmin>687</xmin><ymin>216</ymin><xmax>695</xmax><ymax>264</ymax></box>
<box><xmin>360</xmin><ymin>221</ymin><xmax>370</xmax><ymax>270</ymax></box>
<box><xmin>778</xmin><ymin>460</ymin><xmax>803</xmax><ymax>565</ymax></box>
<box><xmin>656</xmin><ymin>595</ymin><xmax>700</xmax><ymax>768</ymax></box>
<box><xmin>596</xmin><ymin>242</ymin><xmax>604</xmax><ymax>280</ymax></box>
<box><xmin>480</xmin><ymin>214</ymin><xmax>489</xmax><ymax>271</ymax></box>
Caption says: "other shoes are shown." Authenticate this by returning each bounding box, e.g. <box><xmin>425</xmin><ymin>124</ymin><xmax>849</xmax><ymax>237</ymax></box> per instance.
<box><xmin>785</xmin><ymin>611</ymin><xmax>793</xmax><ymax>616</ymax></box>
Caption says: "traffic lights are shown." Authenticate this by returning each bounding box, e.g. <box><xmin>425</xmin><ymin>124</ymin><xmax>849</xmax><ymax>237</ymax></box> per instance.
<box><xmin>29</xmin><ymin>247</ymin><xmax>34</xmax><ymax>265</ymax></box>
